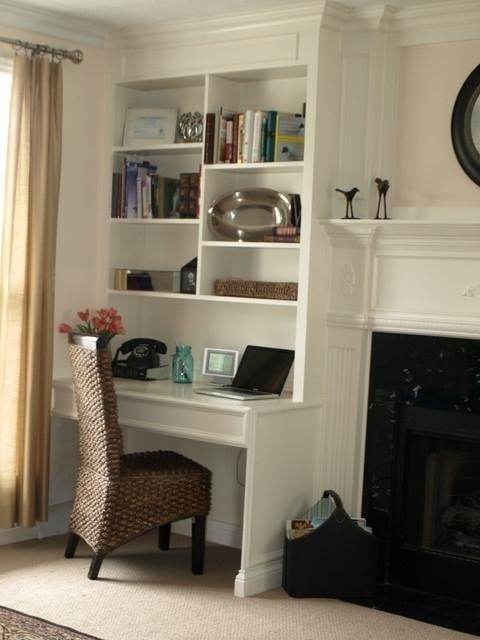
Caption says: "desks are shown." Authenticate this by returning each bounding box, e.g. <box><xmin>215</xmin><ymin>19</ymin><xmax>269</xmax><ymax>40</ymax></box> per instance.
<box><xmin>52</xmin><ymin>375</ymin><xmax>322</xmax><ymax>598</ymax></box>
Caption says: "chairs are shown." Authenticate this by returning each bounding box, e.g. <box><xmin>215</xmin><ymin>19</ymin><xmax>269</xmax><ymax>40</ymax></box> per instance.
<box><xmin>65</xmin><ymin>332</ymin><xmax>213</xmax><ymax>580</ymax></box>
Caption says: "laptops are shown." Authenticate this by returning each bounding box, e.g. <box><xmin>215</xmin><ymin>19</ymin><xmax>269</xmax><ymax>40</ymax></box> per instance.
<box><xmin>196</xmin><ymin>344</ymin><xmax>295</xmax><ymax>402</ymax></box>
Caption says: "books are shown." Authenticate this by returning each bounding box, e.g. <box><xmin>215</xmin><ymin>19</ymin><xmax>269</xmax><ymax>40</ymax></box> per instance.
<box><xmin>112</xmin><ymin>365</ymin><xmax>170</xmax><ymax>380</ymax></box>
<box><xmin>205</xmin><ymin>106</ymin><xmax>305</xmax><ymax>164</ymax></box>
<box><xmin>110</xmin><ymin>154</ymin><xmax>199</xmax><ymax>219</ymax></box>
<box><xmin>286</xmin><ymin>518</ymin><xmax>373</xmax><ymax>540</ymax></box>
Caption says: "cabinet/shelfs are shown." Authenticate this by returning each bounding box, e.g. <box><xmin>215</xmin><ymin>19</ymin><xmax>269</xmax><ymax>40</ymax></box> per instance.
<box><xmin>106</xmin><ymin>61</ymin><xmax>319</xmax><ymax>306</ymax></box>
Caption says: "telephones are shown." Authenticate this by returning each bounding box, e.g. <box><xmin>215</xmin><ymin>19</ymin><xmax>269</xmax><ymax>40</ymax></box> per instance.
<box><xmin>121</xmin><ymin>338</ymin><xmax>167</xmax><ymax>370</ymax></box>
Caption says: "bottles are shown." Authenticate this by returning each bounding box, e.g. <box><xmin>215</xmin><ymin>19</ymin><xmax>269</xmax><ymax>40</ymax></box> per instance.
<box><xmin>171</xmin><ymin>344</ymin><xmax>195</xmax><ymax>383</ymax></box>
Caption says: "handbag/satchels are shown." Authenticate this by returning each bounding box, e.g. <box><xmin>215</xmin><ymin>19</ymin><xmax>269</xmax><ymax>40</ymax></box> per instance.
<box><xmin>281</xmin><ymin>488</ymin><xmax>372</xmax><ymax>599</ymax></box>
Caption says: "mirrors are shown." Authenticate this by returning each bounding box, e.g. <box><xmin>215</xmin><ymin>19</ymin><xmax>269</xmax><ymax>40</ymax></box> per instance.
<box><xmin>450</xmin><ymin>63</ymin><xmax>480</xmax><ymax>189</ymax></box>
